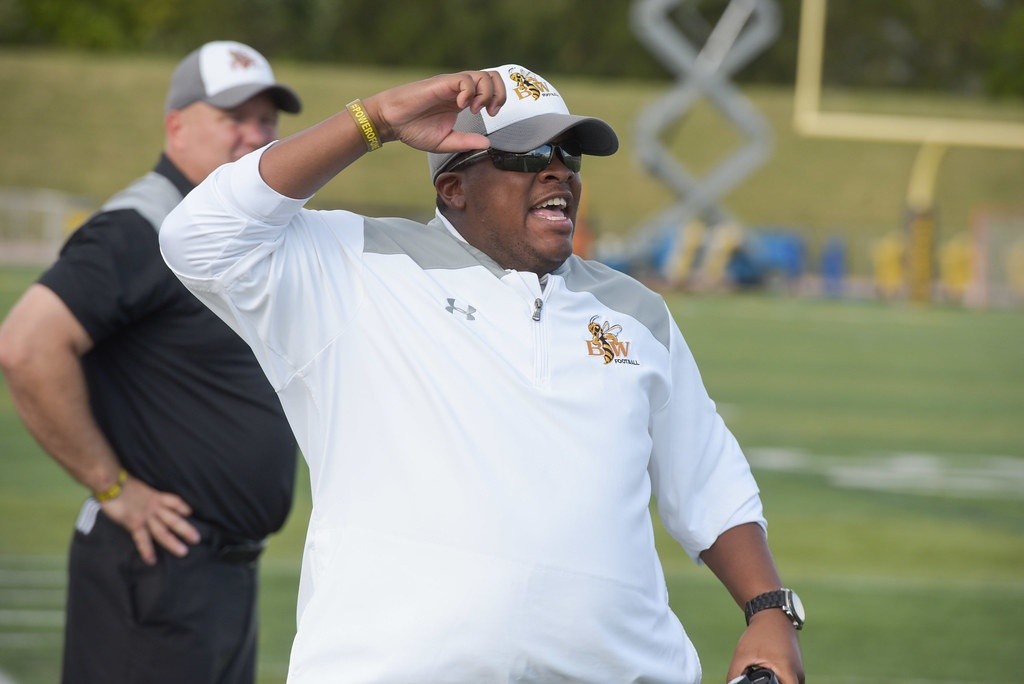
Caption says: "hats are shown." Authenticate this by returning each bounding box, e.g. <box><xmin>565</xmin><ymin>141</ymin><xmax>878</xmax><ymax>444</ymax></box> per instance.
<box><xmin>427</xmin><ymin>64</ymin><xmax>618</xmax><ymax>184</ymax></box>
<box><xmin>165</xmin><ymin>41</ymin><xmax>302</xmax><ymax>117</ymax></box>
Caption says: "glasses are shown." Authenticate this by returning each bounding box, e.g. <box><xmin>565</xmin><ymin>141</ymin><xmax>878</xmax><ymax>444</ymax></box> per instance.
<box><xmin>447</xmin><ymin>139</ymin><xmax>583</xmax><ymax>172</ymax></box>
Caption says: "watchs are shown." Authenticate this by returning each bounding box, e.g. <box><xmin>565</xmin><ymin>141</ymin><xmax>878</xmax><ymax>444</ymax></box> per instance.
<box><xmin>744</xmin><ymin>587</ymin><xmax>806</xmax><ymax>630</ymax></box>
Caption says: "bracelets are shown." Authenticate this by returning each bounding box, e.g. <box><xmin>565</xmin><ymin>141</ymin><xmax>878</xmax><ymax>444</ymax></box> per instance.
<box><xmin>346</xmin><ymin>98</ymin><xmax>383</xmax><ymax>152</ymax></box>
<box><xmin>94</xmin><ymin>468</ymin><xmax>129</xmax><ymax>501</ymax></box>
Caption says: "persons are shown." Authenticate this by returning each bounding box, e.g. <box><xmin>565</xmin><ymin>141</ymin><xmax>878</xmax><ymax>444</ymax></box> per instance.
<box><xmin>1</xmin><ymin>40</ymin><xmax>302</xmax><ymax>684</ymax></box>
<box><xmin>159</xmin><ymin>64</ymin><xmax>806</xmax><ymax>684</ymax></box>
